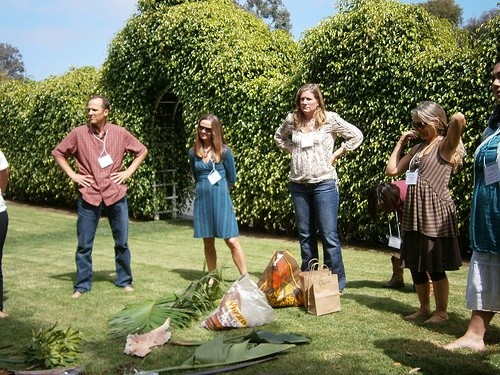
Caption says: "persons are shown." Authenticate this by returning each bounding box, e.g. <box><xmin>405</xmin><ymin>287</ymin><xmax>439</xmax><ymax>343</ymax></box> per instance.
<box><xmin>0</xmin><ymin>150</ymin><xmax>9</xmax><ymax>318</ymax></box>
<box><xmin>368</xmin><ymin>180</ymin><xmax>416</xmax><ymax>293</ymax></box>
<box><xmin>385</xmin><ymin>101</ymin><xmax>466</xmax><ymax>323</ymax></box>
<box><xmin>273</xmin><ymin>85</ymin><xmax>364</xmax><ymax>293</ymax></box>
<box><xmin>188</xmin><ymin>115</ymin><xmax>247</xmax><ymax>276</ymax></box>
<box><xmin>51</xmin><ymin>95</ymin><xmax>148</xmax><ymax>298</ymax></box>
<box><xmin>440</xmin><ymin>54</ymin><xmax>500</xmax><ymax>351</ymax></box>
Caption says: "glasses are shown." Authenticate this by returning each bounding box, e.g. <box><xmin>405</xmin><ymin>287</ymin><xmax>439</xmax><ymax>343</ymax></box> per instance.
<box><xmin>413</xmin><ymin>122</ymin><xmax>427</xmax><ymax>128</ymax></box>
<box><xmin>199</xmin><ymin>125</ymin><xmax>212</xmax><ymax>131</ymax></box>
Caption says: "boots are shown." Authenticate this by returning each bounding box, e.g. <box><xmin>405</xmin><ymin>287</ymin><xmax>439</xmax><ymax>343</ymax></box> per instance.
<box><xmin>382</xmin><ymin>254</ymin><xmax>405</xmax><ymax>289</ymax></box>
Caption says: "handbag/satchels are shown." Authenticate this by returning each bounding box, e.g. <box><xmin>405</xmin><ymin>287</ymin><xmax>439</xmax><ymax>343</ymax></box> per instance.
<box><xmin>198</xmin><ymin>270</ymin><xmax>279</xmax><ymax>330</ymax></box>
<box><xmin>297</xmin><ymin>259</ymin><xmax>326</xmax><ymax>309</ymax></box>
<box><xmin>254</xmin><ymin>249</ymin><xmax>304</xmax><ymax>308</ymax></box>
<box><xmin>307</xmin><ymin>264</ymin><xmax>341</xmax><ymax>316</ymax></box>
